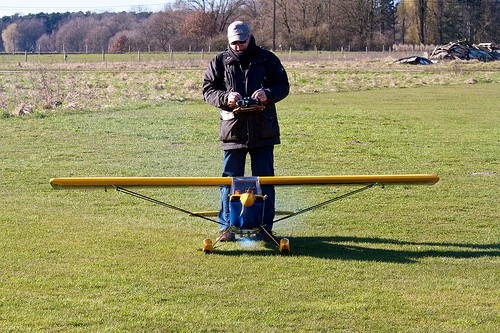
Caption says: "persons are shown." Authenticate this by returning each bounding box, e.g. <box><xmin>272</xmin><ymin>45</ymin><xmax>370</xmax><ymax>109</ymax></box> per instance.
<box><xmin>201</xmin><ymin>21</ymin><xmax>291</xmax><ymax>245</ymax></box>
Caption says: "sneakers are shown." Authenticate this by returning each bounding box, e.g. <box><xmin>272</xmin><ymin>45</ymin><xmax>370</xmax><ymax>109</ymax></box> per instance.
<box><xmin>264</xmin><ymin>236</ymin><xmax>279</xmax><ymax>243</ymax></box>
<box><xmin>216</xmin><ymin>232</ymin><xmax>235</xmax><ymax>242</ymax></box>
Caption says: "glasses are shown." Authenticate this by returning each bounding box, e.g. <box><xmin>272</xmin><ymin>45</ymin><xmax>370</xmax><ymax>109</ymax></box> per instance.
<box><xmin>231</xmin><ymin>37</ymin><xmax>250</xmax><ymax>45</ymax></box>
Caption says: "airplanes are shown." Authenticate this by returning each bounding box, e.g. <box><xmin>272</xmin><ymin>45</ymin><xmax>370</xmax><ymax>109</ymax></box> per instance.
<box><xmin>50</xmin><ymin>174</ymin><xmax>441</xmax><ymax>256</ymax></box>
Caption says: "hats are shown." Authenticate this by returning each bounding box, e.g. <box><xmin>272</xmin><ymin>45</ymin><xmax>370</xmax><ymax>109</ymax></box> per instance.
<box><xmin>226</xmin><ymin>21</ymin><xmax>249</xmax><ymax>45</ymax></box>
